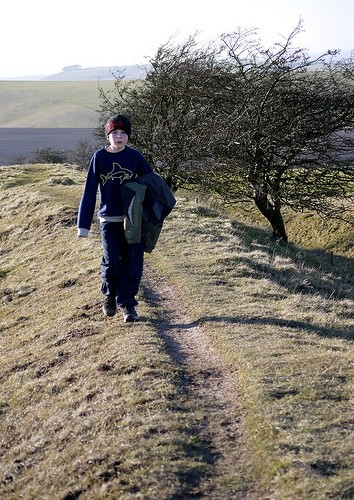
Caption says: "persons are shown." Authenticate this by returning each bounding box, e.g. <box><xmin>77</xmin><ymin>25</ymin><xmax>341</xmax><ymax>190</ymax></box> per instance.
<box><xmin>77</xmin><ymin>114</ymin><xmax>176</xmax><ymax>323</ymax></box>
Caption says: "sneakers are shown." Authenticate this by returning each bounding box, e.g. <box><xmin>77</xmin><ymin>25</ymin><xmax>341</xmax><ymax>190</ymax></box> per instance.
<box><xmin>102</xmin><ymin>295</ymin><xmax>117</xmax><ymax>317</ymax></box>
<box><xmin>121</xmin><ymin>306</ymin><xmax>138</xmax><ymax>322</ymax></box>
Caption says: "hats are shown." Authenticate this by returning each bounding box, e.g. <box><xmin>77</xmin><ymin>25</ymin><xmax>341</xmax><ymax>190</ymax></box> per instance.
<box><xmin>104</xmin><ymin>114</ymin><xmax>131</xmax><ymax>139</ymax></box>
